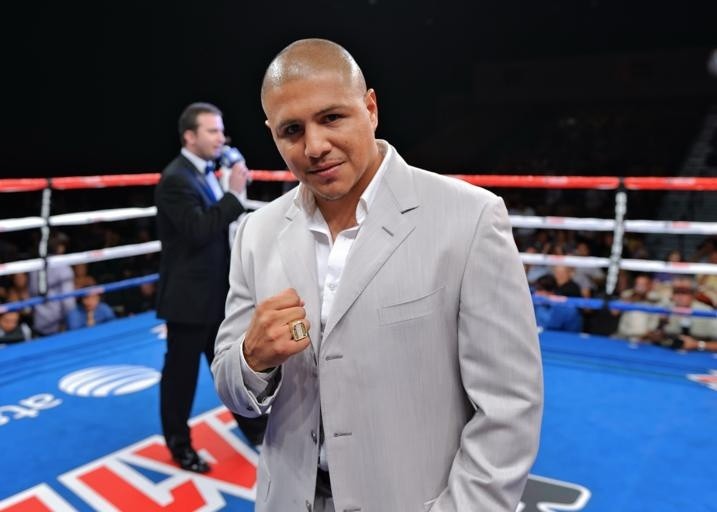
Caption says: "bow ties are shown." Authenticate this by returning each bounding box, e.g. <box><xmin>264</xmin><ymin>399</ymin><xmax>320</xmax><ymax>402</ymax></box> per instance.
<box><xmin>206</xmin><ymin>163</ymin><xmax>216</xmax><ymax>173</ymax></box>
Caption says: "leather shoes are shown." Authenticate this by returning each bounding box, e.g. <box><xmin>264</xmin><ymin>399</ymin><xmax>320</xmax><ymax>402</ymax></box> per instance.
<box><xmin>178</xmin><ymin>446</ymin><xmax>210</xmax><ymax>474</ymax></box>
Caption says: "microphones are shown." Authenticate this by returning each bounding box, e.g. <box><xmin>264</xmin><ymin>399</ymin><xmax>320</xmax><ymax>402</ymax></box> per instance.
<box><xmin>218</xmin><ymin>144</ymin><xmax>253</xmax><ymax>186</ymax></box>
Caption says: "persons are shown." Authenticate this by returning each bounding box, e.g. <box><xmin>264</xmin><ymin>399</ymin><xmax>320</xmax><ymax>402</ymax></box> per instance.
<box><xmin>150</xmin><ymin>100</ymin><xmax>269</xmax><ymax>475</ymax></box>
<box><xmin>200</xmin><ymin>37</ymin><xmax>548</xmax><ymax>511</ymax></box>
<box><xmin>505</xmin><ymin>201</ymin><xmax>717</xmax><ymax>353</ymax></box>
<box><xmin>1</xmin><ymin>217</ymin><xmax>161</xmax><ymax>349</ymax></box>
